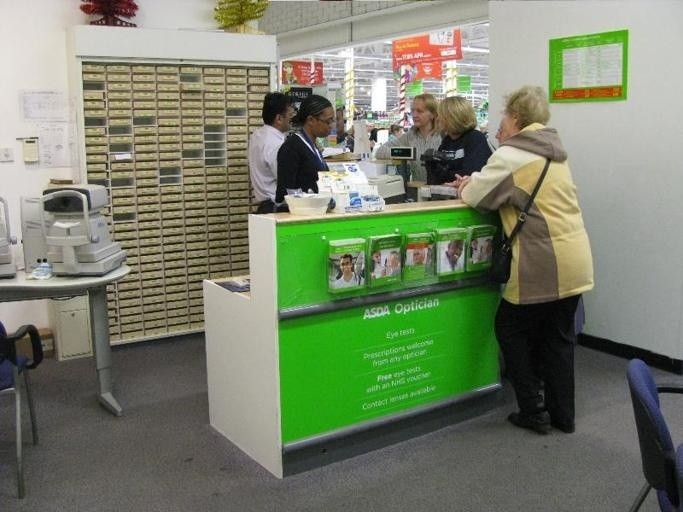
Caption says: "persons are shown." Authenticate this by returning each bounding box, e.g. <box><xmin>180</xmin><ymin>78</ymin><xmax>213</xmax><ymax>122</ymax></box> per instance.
<box><xmin>494</xmin><ymin>116</ymin><xmax>507</xmax><ymax>148</ymax></box>
<box><xmin>273</xmin><ymin>95</ymin><xmax>335</xmax><ymax>212</ymax></box>
<box><xmin>374</xmin><ymin>94</ymin><xmax>445</xmax><ymax>182</ymax></box>
<box><xmin>247</xmin><ymin>91</ymin><xmax>294</xmax><ymax>214</ymax></box>
<box><xmin>442</xmin><ymin>85</ymin><xmax>594</xmax><ymax>435</ymax></box>
<box><xmin>287</xmin><ymin>104</ymin><xmax>298</xmax><ymax>137</ymax></box>
<box><xmin>425</xmin><ymin>96</ymin><xmax>497</xmax><ymax>187</ymax></box>
<box><xmin>335</xmin><ymin>108</ymin><xmax>404</xmax><ymax>154</ymax></box>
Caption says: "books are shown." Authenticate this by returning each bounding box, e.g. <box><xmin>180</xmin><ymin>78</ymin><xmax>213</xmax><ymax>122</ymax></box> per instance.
<box><xmin>327</xmin><ymin>222</ymin><xmax>497</xmax><ymax>296</ymax></box>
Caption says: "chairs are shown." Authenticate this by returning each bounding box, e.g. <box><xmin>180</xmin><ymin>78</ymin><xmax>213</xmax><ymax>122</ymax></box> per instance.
<box><xmin>0</xmin><ymin>321</ymin><xmax>44</xmax><ymax>499</ymax></box>
<box><xmin>626</xmin><ymin>359</ymin><xmax>683</xmax><ymax>512</ymax></box>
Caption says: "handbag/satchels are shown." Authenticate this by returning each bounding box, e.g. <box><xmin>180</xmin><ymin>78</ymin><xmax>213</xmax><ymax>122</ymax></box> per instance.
<box><xmin>490</xmin><ymin>226</ymin><xmax>512</xmax><ymax>282</ymax></box>
<box><xmin>419</xmin><ymin>148</ymin><xmax>448</xmax><ymax>185</ymax></box>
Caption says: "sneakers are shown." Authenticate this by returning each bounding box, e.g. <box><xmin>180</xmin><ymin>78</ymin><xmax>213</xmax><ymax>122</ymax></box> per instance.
<box><xmin>508</xmin><ymin>411</ymin><xmax>552</xmax><ymax>432</ymax></box>
<box><xmin>553</xmin><ymin>418</ymin><xmax>574</xmax><ymax>432</ymax></box>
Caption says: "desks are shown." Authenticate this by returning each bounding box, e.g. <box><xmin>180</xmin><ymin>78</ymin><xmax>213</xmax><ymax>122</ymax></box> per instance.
<box><xmin>0</xmin><ymin>263</ymin><xmax>131</xmax><ymax>416</ymax></box>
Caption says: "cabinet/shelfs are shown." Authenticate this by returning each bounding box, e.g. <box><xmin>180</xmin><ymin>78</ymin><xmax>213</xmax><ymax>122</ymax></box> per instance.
<box><xmin>65</xmin><ymin>24</ymin><xmax>280</xmax><ymax>348</ymax></box>
<box><xmin>353</xmin><ymin>119</ymin><xmax>398</xmax><ymax>154</ymax></box>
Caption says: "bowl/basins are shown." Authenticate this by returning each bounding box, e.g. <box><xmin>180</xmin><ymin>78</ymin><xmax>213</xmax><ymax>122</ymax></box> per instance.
<box><xmin>283</xmin><ymin>192</ymin><xmax>332</xmax><ymax>216</ymax></box>
